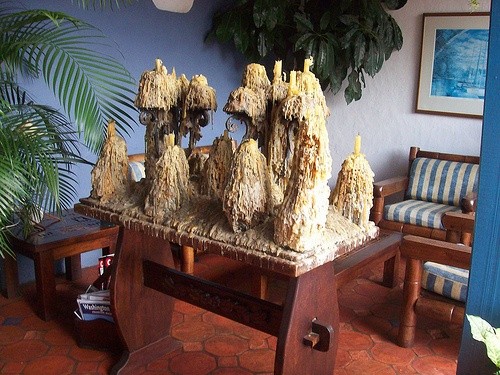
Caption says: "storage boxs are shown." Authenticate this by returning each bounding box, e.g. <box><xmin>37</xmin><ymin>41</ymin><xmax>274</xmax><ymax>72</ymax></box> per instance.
<box><xmin>73</xmin><ymin>317</ymin><xmax>124</xmax><ymax>348</ymax></box>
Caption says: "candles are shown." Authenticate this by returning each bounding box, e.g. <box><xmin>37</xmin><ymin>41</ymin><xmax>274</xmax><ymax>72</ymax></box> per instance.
<box><xmin>165</xmin><ymin>132</ymin><xmax>174</xmax><ymax>147</ymax></box>
<box><xmin>273</xmin><ymin>59</ymin><xmax>282</xmax><ymax>79</ymax></box>
<box><xmin>156</xmin><ymin>56</ymin><xmax>163</xmax><ymax>74</ymax></box>
<box><xmin>354</xmin><ymin>132</ymin><xmax>363</xmax><ymax>156</ymax></box>
<box><xmin>107</xmin><ymin>119</ymin><xmax>115</xmax><ymax>138</ymax></box>
<box><xmin>304</xmin><ymin>58</ymin><xmax>310</xmax><ymax>72</ymax></box>
<box><xmin>223</xmin><ymin>130</ymin><xmax>228</xmax><ymax>143</ymax></box>
<box><xmin>287</xmin><ymin>71</ymin><xmax>298</xmax><ymax>97</ymax></box>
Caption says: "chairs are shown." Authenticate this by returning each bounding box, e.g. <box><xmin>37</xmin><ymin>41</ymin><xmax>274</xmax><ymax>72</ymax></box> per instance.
<box><xmin>398</xmin><ymin>211</ymin><xmax>476</xmax><ymax>348</ymax></box>
<box><xmin>370</xmin><ymin>147</ymin><xmax>480</xmax><ymax>246</ymax></box>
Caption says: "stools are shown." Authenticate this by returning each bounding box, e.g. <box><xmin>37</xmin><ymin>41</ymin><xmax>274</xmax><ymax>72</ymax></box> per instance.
<box><xmin>1</xmin><ymin>204</ymin><xmax>120</xmax><ymax>322</ymax></box>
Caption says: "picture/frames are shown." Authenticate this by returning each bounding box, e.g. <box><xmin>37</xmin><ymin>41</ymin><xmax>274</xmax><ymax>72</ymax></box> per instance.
<box><xmin>415</xmin><ymin>12</ymin><xmax>490</xmax><ymax>118</ymax></box>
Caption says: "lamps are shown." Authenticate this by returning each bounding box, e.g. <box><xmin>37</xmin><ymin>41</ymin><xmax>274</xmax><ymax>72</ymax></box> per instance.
<box><xmin>152</xmin><ymin>0</ymin><xmax>194</xmax><ymax>13</ymax></box>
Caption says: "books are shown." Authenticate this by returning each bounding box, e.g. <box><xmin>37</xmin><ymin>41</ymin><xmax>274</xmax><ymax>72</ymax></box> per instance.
<box><xmin>74</xmin><ymin>284</ymin><xmax>114</xmax><ymax>323</ymax></box>
<box><xmin>98</xmin><ymin>254</ymin><xmax>115</xmax><ymax>276</ymax></box>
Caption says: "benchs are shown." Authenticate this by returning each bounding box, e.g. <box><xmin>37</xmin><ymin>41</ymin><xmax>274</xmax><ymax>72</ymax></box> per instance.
<box><xmin>128</xmin><ymin>146</ymin><xmax>216</xmax><ymax>182</ymax></box>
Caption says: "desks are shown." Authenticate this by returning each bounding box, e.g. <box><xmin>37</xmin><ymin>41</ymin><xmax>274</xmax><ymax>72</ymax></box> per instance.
<box><xmin>74</xmin><ymin>199</ymin><xmax>403</xmax><ymax>375</ymax></box>
